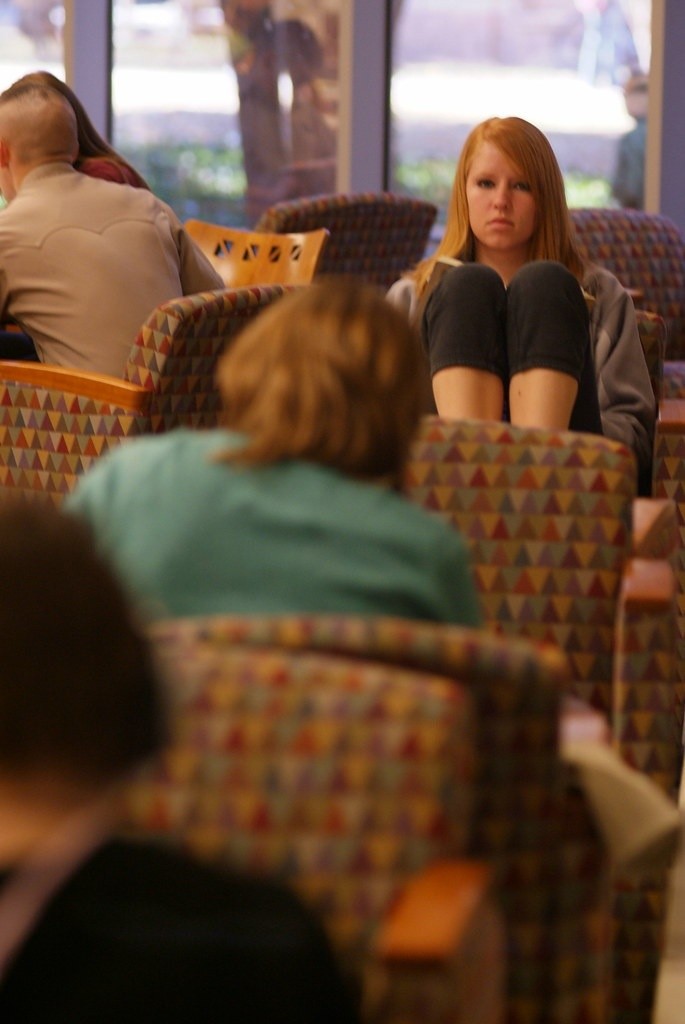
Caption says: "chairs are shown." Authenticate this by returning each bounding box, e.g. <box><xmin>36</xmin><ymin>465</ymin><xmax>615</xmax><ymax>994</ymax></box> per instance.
<box><xmin>0</xmin><ymin>193</ymin><xmax>684</xmax><ymax>1024</ymax></box>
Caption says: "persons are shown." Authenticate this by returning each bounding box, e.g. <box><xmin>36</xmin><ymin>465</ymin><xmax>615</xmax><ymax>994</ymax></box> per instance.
<box><xmin>386</xmin><ymin>116</ymin><xmax>655</xmax><ymax>478</ymax></box>
<box><xmin>0</xmin><ymin>83</ymin><xmax>225</xmax><ymax>380</ymax></box>
<box><xmin>609</xmin><ymin>75</ymin><xmax>648</xmax><ymax>210</ymax></box>
<box><xmin>11</xmin><ymin>70</ymin><xmax>151</xmax><ymax>190</ymax></box>
<box><xmin>63</xmin><ymin>279</ymin><xmax>482</xmax><ymax>631</ymax></box>
<box><xmin>0</xmin><ymin>493</ymin><xmax>358</xmax><ymax>1024</ymax></box>
